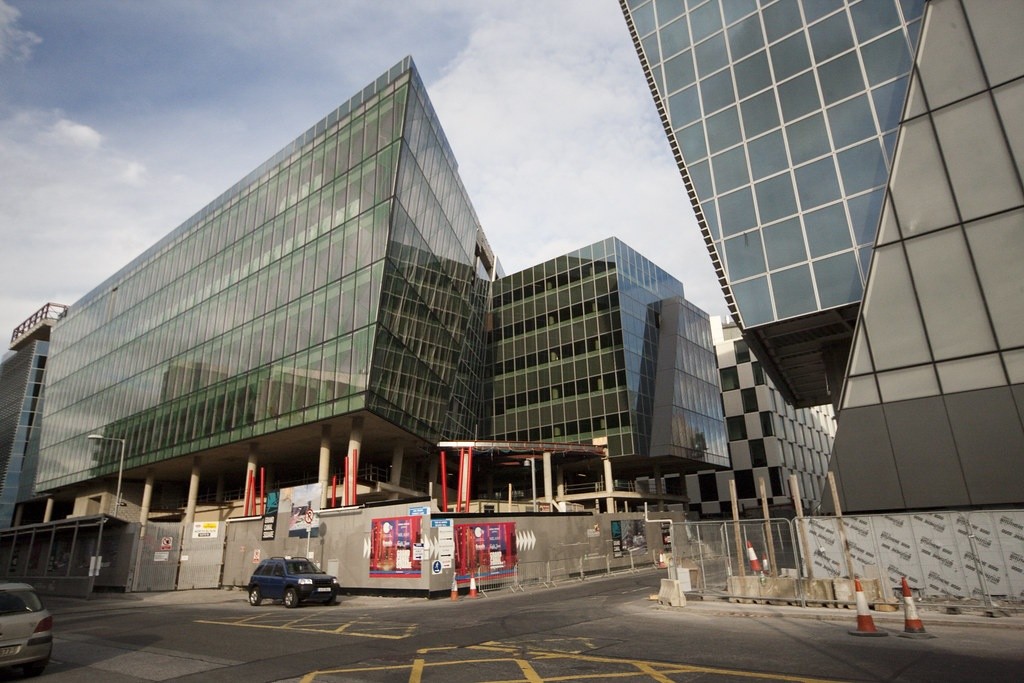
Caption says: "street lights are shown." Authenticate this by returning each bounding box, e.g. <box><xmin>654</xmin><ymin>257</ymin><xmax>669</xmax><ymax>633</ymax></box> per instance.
<box><xmin>524</xmin><ymin>456</ymin><xmax>537</xmax><ymax>512</ymax></box>
<box><xmin>88</xmin><ymin>434</ymin><xmax>126</xmax><ymax>518</ymax></box>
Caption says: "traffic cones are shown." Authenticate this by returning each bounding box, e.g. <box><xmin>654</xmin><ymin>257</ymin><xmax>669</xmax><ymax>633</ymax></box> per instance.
<box><xmin>847</xmin><ymin>575</ymin><xmax>889</xmax><ymax>636</ymax></box>
<box><xmin>744</xmin><ymin>540</ymin><xmax>770</xmax><ymax>577</ymax></box>
<box><xmin>464</xmin><ymin>571</ymin><xmax>483</xmax><ymax>599</ymax></box>
<box><xmin>760</xmin><ymin>549</ymin><xmax>773</xmax><ymax>576</ymax></box>
<box><xmin>899</xmin><ymin>576</ymin><xmax>938</xmax><ymax>639</ymax></box>
<box><xmin>450</xmin><ymin>580</ymin><xmax>459</xmax><ymax>601</ymax></box>
<box><xmin>658</xmin><ymin>549</ymin><xmax>668</xmax><ymax>569</ymax></box>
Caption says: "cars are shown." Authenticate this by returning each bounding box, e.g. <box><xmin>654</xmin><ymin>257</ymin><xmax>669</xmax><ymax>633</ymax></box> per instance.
<box><xmin>0</xmin><ymin>582</ymin><xmax>54</xmax><ymax>677</ymax></box>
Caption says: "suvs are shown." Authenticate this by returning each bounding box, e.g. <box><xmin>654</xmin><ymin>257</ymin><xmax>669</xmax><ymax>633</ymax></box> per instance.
<box><xmin>248</xmin><ymin>556</ymin><xmax>340</xmax><ymax>609</ymax></box>
